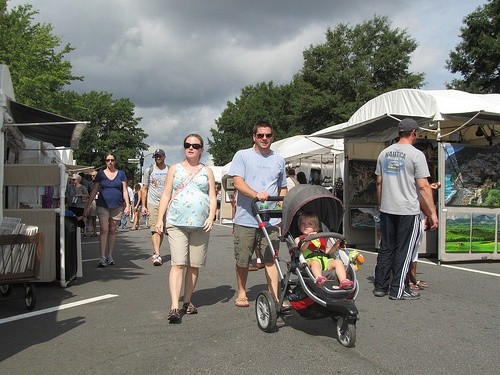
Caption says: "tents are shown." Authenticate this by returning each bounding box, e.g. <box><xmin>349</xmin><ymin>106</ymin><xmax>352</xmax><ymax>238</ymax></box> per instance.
<box><xmin>303</xmin><ymin>88</ymin><xmax>500</xmax><ymax>263</ymax></box>
<box><xmin>270</xmin><ymin>135</ymin><xmax>344</xmax><ymax>197</ymax></box>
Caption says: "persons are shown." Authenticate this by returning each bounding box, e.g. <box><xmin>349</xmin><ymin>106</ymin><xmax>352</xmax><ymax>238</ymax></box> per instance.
<box><xmin>229</xmin><ymin>123</ymin><xmax>287</xmax><ymax>306</ymax></box>
<box><xmin>287</xmin><ymin>169</ymin><xmax>307</xmax><ymax>189</ymax></box>
<box><xmin>374</xmin><ymin>119</ymin><xmax>439</xmax><ymax>300</ymax></box>
<box><xmin>83</xmin><ymin>152</ymin><xmax>130</xmax><ymax>267</ymax></box>
<box><xmin>120</xmin><ymin>185</ymin><xmax>142</xmax><ymax>230</ymax></box>
<box><xmin>215</xmin><ymin>186</ymin><xmax>221</xmax><ymax>222</ymax></box>
<box><xmin>70</xmin><ymin>171</ymin><xmax>98</xmax><ymax>238</ymax></box>
<box><xmin>309</xmin><ymin>169</ymin><xmax>322</xmax><ymax>186</ymax></box>
<box><xmin>408</xmin><ymin>182</ymin><xmax>442</xmax><ymax>290</ymax></box>
<box><xmin>295</xmin><ymin>212</ymin><xmax>353</xmax><ymax>289</ymax></box>
<box><xmin>155</xmin><ymin>134</ymin><xmax>217</xmax><ymax>320</ymax></box>
<box><xmin>142</xmin><ymin>149</ymin><xmax>171</xmax><ymax>266</ymax></box>
<box><xmin>55</xmin><ymin>198</ymin><xmax>83</xmax><ymax>220</ymax></box>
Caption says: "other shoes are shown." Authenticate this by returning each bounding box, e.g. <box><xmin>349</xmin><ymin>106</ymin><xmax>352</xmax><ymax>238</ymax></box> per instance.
<box><xmin>99</xmin><ymin>257</ymin><xmax>107</xmax><ymax>266</ymax></box>
<box><xmin>408</xmin><ymin>280</ymin><xmax>430</xmax><ymax>290</ymax></box>
<box><xmin>90</xmin><ymin>233</ymin><xmax>98</xmax><ymax>238</ymax></box>
<box><xmin>248</xmin><ymin>266</ymin><xmax>259</xmax><ymax>270</ymax></box>
<box><xmin>167</xmin><ymin>309</ymin><xmax>183</xmax><ymax>323</ymax></box>
<box><xmin>107</xmin><ymin>255</ymin><xmax>115</xmax><ymax>264</ymax></box>
<box><xmin>181</xmin><ymin>302</ymin><xmax>197</xmax><ymax>314</ymax></box>
<box><xmin>152</xmin><ymin>253</ymin><xmax>163</xmax><ymax>266</ymax></box>
<box><xmin>374</xmin><ymin>291</ymin><xmax>385</xmax><ymax>297</ymax></box>
<box><xmin>340</xmin><ymin>278</ymin><xmax>353</xmax><ymax>289</ymax></box>
<box><xmin>314</xmin><ymin>275</ymin><xmax>327</xmax><ymax>286</ymax></box>
<box><xmin>82</xmin><ymin>233</ymin><xmax>87</xmax><ymax>239</ymax></box>
<box><xmin>256</xmin><ymin>263</ymin><xmax>265</xmax><ymax>269</ymax></box>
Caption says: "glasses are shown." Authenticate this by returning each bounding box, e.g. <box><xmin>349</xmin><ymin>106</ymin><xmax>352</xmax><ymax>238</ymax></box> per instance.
<box><xmin>184</xmin><ymin>142</ymin><xmax>202</xmax><ymax>150</ymax></box>
<box><xmin>257</xmin><ymin>133</ymin><xmax>271</xmax><ymax>138</ymax></box>
<box><xmin>106</xmin><ymin>159</ymin><xmax>115</xmax><ymax>162</ymax></box>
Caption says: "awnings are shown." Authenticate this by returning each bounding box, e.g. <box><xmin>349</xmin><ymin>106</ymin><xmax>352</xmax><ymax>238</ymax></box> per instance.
<box><xmin>3</xmin><ymin>100</ymin><xmax>91</xmax><ymax>150</ymax></box>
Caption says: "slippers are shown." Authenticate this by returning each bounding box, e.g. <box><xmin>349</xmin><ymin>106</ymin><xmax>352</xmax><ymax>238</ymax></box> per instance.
<box><xmin>235</xmin><ymin>294</ymin><xmax>249</xmax><ymax>307</ymax></box>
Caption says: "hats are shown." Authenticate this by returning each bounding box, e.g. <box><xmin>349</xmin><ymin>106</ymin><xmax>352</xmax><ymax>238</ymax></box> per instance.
<box><xmin>154</xmin><ymin>149</ymin><xmax>165</xmax><ymax>156</ymax></box>
<box><xmin>397</xmin><ymin>118</ymin><xmax>418</xmax><ymax>132</ymax></box>
<box><xmin>71</xmin><ymin>174</ymin><xmax>83</xmax><ymax>181</ymax></box>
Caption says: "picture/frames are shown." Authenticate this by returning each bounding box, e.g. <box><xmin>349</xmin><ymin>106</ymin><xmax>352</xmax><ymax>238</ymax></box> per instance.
<box><xmin>225</xmin><ymin>177</ymin><xmax>235</xmax><ymax>189</ymax></box>
<box><xmin>225</xmin><ymin>189</ymin><xmax>235</xmax><ymax>202</ymax></box>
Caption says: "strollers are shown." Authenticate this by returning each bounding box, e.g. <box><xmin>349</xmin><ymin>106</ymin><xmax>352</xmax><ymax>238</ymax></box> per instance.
<box><xmin>254</xmin><ymin>183</ymin><xmax>360</xmax><ymax>348</ymax></box>
